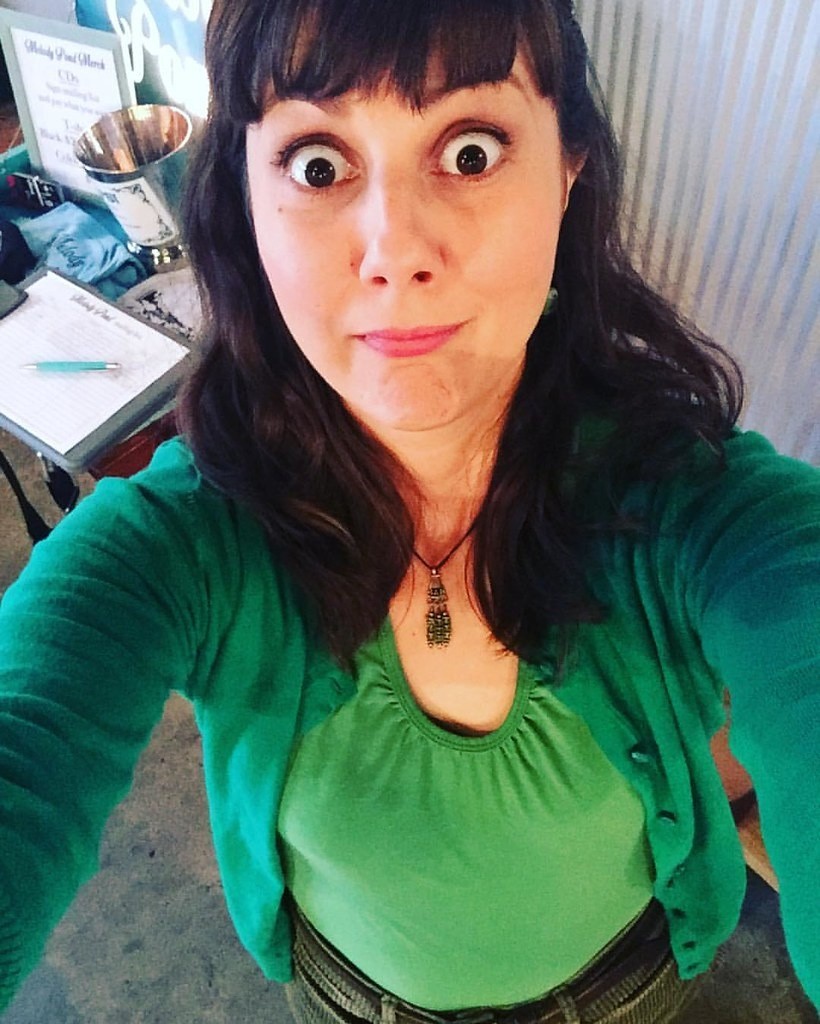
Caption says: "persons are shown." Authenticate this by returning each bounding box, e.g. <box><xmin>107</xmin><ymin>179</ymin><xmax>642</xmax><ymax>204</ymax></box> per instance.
<box><xmin>2</xmin><ymin>1</ymin><xmax>820</xmax><ymax>1024</ymax></box>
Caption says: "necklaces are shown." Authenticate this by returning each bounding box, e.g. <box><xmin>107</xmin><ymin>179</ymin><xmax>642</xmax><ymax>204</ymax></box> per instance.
<box><xmin>408</xmin><ymin>511</ymin><xmax>476</xmax><ymax>650</ymax></box>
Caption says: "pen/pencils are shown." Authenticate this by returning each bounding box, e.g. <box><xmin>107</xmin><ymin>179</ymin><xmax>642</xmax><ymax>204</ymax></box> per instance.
<box><xmin>21</xmin><ymin>361</ymin><xmax>122</xmax><ymax>371</ymax></box>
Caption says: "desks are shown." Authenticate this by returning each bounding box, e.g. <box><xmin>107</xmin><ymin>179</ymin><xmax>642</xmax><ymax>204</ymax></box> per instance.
<box><xmin>1</xmin><ymin>147</ymin><xmax>196</xmax><ymax>482</ymax></box>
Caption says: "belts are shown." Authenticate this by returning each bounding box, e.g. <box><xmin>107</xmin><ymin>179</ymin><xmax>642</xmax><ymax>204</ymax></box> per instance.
<box><xmin>291</xmin><ymin>897</ymin><xmax>670</xmax><ymax>1024</ymax></box>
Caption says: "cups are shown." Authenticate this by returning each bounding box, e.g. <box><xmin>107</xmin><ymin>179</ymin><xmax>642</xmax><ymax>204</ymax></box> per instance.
<box><xmin>74</xmin><ymin>105</ymin><xmax>192</xmax><ymax>273</ymax></box>
